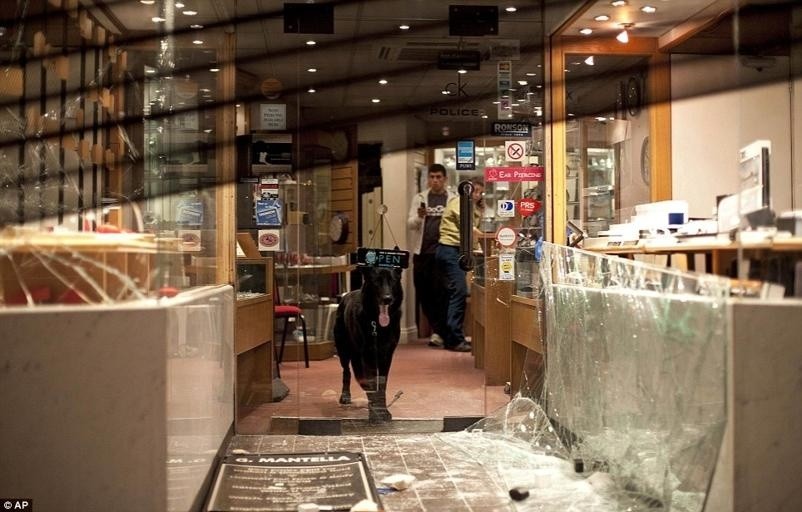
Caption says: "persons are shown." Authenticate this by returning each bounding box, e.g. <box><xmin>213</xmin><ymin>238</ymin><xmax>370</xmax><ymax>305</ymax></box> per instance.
<box><xmin>434</xmin><ymin>176</ymin><xmax>486</xmax><ymax>353</ymax></box>
<box><xmin>406</xmin><ymin>162</ymin><xmax>451</xmax><ymax>349</ymax></box>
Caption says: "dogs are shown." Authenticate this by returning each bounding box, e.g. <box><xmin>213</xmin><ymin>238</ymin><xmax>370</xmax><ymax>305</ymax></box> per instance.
<box><xmin>334</xmin><ymin>259</ymin><xmax>403</xmax><ymax>424</ymax></box>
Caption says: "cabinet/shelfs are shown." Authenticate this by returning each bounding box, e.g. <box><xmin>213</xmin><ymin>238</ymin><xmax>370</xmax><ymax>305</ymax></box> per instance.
<box><xmin>0</xmin><ymin>142</ymin><xmax>357</xmax><ymax>512</ymax></box>
<box><xmin>417</xmin><ymin>111</ymin><xmax>802</xmax><ymax>512</ymax></box>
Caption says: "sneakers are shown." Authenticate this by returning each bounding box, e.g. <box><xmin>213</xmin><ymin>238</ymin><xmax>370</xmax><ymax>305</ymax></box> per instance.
<box><xmin>428</xmin><ymin>332</ymin><xmax>473</xmax><ymax>352</ymax></box>
